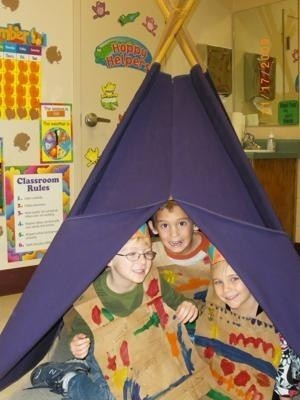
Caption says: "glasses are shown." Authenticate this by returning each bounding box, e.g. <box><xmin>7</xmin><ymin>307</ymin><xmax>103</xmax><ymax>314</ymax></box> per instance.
<box><xmin>116</xmin><ymin>250</ymin><xmax>157</xmax><ymax>262</ymax></box>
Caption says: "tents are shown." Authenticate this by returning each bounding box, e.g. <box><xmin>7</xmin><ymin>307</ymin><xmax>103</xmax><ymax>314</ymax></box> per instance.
<box><xmin>0</xmin><ymin>66</ymin><xmax>300</xmax><ymax>400</ymax></box>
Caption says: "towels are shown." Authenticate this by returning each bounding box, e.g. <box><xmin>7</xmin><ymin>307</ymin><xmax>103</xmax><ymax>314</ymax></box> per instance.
<box><xmin>206</xmin><ymin>44</ymin><xmax>232</xmax><ymax>97</ymax></box>
<box><xmin>250</xmin><ymin>53</ymin><xmax>276</xmax><ymax>100</ymax></box>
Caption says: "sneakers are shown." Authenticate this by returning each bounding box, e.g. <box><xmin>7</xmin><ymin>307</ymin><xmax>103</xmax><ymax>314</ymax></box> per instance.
<box><xmin>30</xmin><ymin>359</ymin><xmax>91</xmax><ymax>397</ymax></box>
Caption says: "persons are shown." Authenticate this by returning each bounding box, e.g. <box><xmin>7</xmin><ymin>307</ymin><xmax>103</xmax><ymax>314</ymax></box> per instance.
<box><xmin>147</xmin><ymin>200</ymin><xmax>212</xmax><ymax>311</ymax></box>
<box><xmin>211</xmin><ymin>260</ymin><xmax>263</xmax><ymax>321</ymax></box>
<box><xmin>29</xmin><ymin>229</ymin><xmax>199</xmax><ymax>400</ymax></box>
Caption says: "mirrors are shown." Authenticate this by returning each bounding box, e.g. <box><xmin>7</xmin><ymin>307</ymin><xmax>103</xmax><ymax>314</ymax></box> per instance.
<box><xmin>231</xmin><ymin>0</ymin><xmax>299</xmax><ymax>128</ymax></box>
